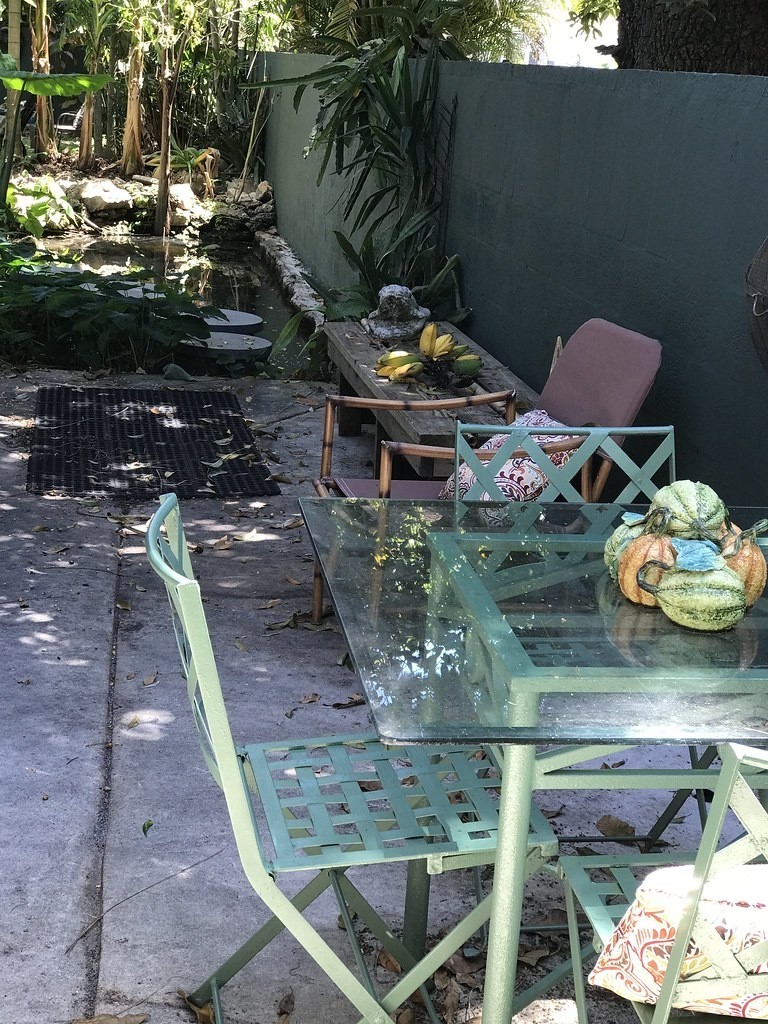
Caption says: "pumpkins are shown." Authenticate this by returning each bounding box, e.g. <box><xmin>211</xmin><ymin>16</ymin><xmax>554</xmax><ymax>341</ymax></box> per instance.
<box><xmin>599</xmin><ymin>580</ymin><xmax>760</xmax><ymax>689</ymax></box>
<box><xmin>604</xmin><ymin>479</ymin><xmax>768</xmax><ymax>631</ymax></box>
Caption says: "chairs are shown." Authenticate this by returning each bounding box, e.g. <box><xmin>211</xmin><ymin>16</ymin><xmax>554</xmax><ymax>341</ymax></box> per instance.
<box><xmin>144</xmin><ymin>492</ymin><xmax>558</xmax><ymax>1024</ymax></box>
<box><xmin>453</xmin><ymin>420</ymin><xmax>710</xmax><ymax>962</ymax></box>
<box><xmin>559</xmin><ymin>744</ymin><xmax>767</xmax><ymax>1024</ymax></box>
<box><xmin>311</xmin><ymin>318</ymin><xmax>663</xmax><ymax>630</ymax></box>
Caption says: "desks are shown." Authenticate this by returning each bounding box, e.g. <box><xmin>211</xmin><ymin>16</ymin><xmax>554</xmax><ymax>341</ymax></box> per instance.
<box><xmin>325</xmin><ymin>321</ymin><xmax>540</xmax><ymax>477</ymax></box>
<box><xmin>296</xmin><ymin>496</ymin><xmax>767</xmax><ymax>1024</ymax></box>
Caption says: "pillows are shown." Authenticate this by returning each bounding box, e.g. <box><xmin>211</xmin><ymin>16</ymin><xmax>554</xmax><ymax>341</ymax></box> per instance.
<box><xmin>586</xmin><ymin>861</ymin><xmax>768</xmax><ymax>1020</ymax></box>
<box><xmin>438</xmin><ymin>409</ymin><xmax>581</xmax><ymax>501</ymax></box>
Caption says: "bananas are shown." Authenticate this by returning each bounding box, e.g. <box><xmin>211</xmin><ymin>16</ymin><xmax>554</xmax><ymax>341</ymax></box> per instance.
<box><xmin>373</xmin><ymin>321</ymin><xmax>485</xmax><ymax>387</ymax></box>
